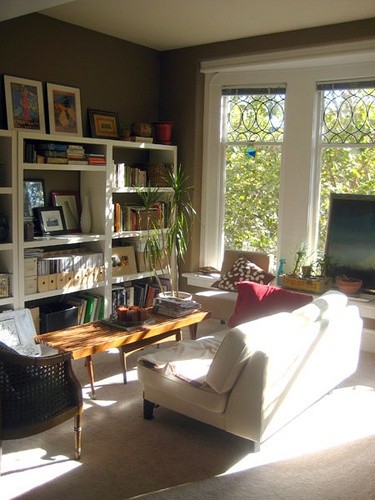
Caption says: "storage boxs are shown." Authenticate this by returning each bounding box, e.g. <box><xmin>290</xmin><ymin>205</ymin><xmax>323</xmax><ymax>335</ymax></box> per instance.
<box><xmin>29</xmin><ymin>307</ymin><xmax>39</xmax><ymax>334</ymax></box>
<box><xmin>40</xmin><ymin>303</ymin><xmax>78</xmax><ymax>333</ymax></box>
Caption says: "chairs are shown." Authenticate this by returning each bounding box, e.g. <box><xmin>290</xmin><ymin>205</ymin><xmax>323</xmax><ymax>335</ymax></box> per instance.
<box><xmin>1</xmin><ymin>342</ymin><xmax>83</xmax><ymax>459</ymax></box>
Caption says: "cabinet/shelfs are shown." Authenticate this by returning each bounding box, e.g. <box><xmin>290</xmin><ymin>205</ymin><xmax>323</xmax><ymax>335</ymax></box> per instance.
<box><xmin>315</xmin><ymin>295</ymin><xmax>375</xmax><ymax>353</ymax></box>
<box><xmin>17</xmin><ymin>128</ymin><xmax>110</xmax><ymax>334</ymax></box>
<box><xmin>0</xmin><ymin>131</ymin><xmax>19</xmax><ymax>312</ymax></box>
<box><xmin>112</xmin><ymin>140</ymin><xmax>177</xmax><ymax>317</ymax></box>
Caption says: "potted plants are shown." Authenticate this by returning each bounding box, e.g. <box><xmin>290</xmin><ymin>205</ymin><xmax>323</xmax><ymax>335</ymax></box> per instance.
<box><xmin>336</xmin><ymin>270</ymin><xmax>362</xmax><ymax>294</ymax></box>
<box><xmin>278</xmin><ymin>246</ymin><xmax>333</xmax><ymax>293</ymax></box>
<box><xmin>131</xmin><ymin>164</ymin><xmax>202</xmax><ymax>317</ymax></box>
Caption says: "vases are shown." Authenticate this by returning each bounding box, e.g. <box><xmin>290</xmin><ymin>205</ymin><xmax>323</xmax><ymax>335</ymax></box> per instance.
<box><xmin>277</xmin><ymin>258</ymin><xmax>286</xmax><ymax>285</ymax></box>
<box><xmin>153</xmin><ymin>121</ymin><xmax>177</xmax><ymax>144</ymax></box>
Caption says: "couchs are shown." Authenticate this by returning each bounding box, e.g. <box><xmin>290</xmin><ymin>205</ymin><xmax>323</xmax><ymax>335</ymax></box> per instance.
<box><xmin>137</xmin><ymin>290</ymin><xmax>346</xmax><ymax>453</ymax></box>
<box><xmin>194</xmin><ymin>249</ymin><xmax>273</xmax><ymax>324</ymax></box>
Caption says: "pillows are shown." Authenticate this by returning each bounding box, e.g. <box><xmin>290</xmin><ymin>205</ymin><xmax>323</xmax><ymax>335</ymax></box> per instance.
<box><xmin>228</xmin><ymin>280</ymin><xmax>312</xmax><ymax>329</ymax></box>
<box><xmin>210</xmin><ymin>254</ymin><xmax>275</xmax><ymax>292</ymax></box>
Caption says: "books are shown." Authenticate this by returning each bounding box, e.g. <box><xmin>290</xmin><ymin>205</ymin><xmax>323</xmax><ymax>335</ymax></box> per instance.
<box><xmin>24</xmin><ymin>248</ymin><xmax>105</xmax><ymax>294</ymax></box>
<box><xmin>112</xmin><ymin>160</ymin><xmax>146</xmax><ymax>186</ymax></box>
<box><xmin>27</xmin><ymin>143</ymin><xmax>106</xmax><ymax>165</ymax></box>
<box><xmin>30</xmin><ymin>292</ymin><xmax>105</xmax><ymax>326</ymax></box>
<box><xmin>115</xmin><ymin>201</ymin><xmax>167</xmax><ymax>231</ymax></box>
<box><xmin>112</xmin><ymin>280</ymin><xmax>166</xmax><ymax>316</ymax></box>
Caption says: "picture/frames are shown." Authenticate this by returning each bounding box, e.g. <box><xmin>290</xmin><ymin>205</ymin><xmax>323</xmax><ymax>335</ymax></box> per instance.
<box><xmin>32</xmin><ymin>206</ymin><xmax>67</xmax><ymax>236</ymax></box>
<box><xmin>88</xmin><ymin>109</ymin><xmax>120</xmax><ymax>138</ymax></box>
<box><xmin>23</xmin><ymin>178</ymin><xmax>46</xmax><ymax>216</ymax></box>
<box><xmin>49</xmin><ymin>192</ymin><xmax>81</xmax><ymax>232</ymax></box>
<box><xmin>45</xmin><ymin>82</ymin><xmax>84</xmax><ymax>138</ymax></box>
<box><xmin>3</xmin><ymin>74</ymin><xmax>46</xmax><ymax>134</ymax></box>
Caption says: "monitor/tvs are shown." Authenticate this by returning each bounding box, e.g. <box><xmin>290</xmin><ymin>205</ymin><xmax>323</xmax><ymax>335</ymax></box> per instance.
<box><xmin>324</xmin><ymin>192</ymin><xmax>375</xmax><ymax>286</ymax></box>
